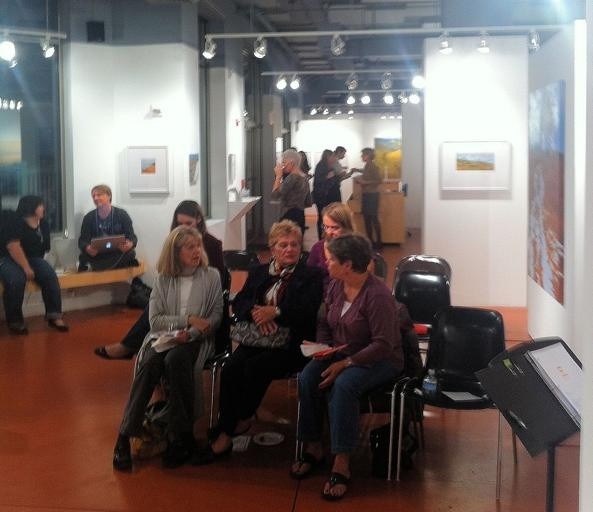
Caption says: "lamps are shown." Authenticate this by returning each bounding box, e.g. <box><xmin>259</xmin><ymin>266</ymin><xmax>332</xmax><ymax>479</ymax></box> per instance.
<box><xmin>0</xmin><ymin>96</ymin><xmax>24</xmax><ymax>112</ymax></box>
<box><xmin>202</xmin><ymin>27</ymin><xmax>541</xmax><ymax>60</ymax></box>
<box><xmin>0</xmin><ymin>24</ymin><xmax>67</xmax><ymax>63</ymax></box>
<box><xmin>261</xmin><ymin>69</ymin><xmax>424</xmax><ymax>121</ymax></box>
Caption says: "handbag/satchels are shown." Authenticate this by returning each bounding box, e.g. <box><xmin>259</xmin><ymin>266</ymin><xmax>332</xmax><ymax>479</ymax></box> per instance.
<box><xmin>229</xmin><ymin>315</ymin><xmax>288</xmax><ymax>348</ymax></box>
<box><xmin>369</xmin><ymin>425</ymin><xmax>405</xmax><ymax>464</ymax></box>
<box><xmin>126</xmin><ymin>278</ymin><xmax>152</xmax><ymax>309</ymax></box>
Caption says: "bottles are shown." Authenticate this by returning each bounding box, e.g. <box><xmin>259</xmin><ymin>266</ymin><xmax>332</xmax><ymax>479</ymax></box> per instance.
<box><xmin>420</xmin><ymin>368</ymin><xmax>437</xmax><ymax>397</ymax></box>
<box><xmin>412</xmin><ymin>326</ymin><xmax>430</xmax><ymax>368</ymax></box>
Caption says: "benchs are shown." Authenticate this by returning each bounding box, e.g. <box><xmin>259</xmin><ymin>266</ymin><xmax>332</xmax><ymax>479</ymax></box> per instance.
<box><xmin>1</xmin><ymin>262</ymin><xmax>146</xmax><ymax>295</ymax></box>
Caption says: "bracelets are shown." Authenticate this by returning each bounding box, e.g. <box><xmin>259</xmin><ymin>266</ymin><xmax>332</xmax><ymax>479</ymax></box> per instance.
<box><xmin>343</xmin><ymin>356</ymin><xmax>351</xmax><ymax>369</ymax></box>
<box><xmin>276</xmin><ymin>175</ymin><xmax>282</xmax><ymax>180</ymax></box>
<box><xmin>274</xmin><ymin>305</ymin><xmax>280</xmax><ymax>317</ymax></box>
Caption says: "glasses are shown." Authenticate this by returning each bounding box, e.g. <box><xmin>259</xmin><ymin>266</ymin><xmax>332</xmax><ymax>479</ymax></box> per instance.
<box><xmin>322</xmin><ymin>224</ymin><xmax>340</xmax><ymax>231</ymax></box>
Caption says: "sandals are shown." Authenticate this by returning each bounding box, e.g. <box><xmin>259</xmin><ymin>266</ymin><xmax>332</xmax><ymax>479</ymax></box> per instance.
<box><xmin>322</xmin><ymin>471</ymin><xmax>349</xmax><ymax>502</ymax></box>
<box><xmin>291</xmin><ymin>454</ymin><xmax>318</xmax><ymax>480</ymax></box>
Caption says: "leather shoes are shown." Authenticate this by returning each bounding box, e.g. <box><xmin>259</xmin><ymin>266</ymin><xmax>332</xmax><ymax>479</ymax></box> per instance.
<box><xmin>14</xmin><ymin>327</ymin><xmax>27</xmax><ymax>335</ymax></box>
<box><xmin>94</xmin><ymin>346</ymin><xmax>132</xmax><ymax>360</ymax></box>
<box><xmin>210</xmin><ymin>424</ymin><xmax>250</xmax><ymax>439</ymax></box>
<box><xmin>203</xmin><ymin>439</ymin><xmax>233</xmax><ymax>466</ymax></box>
<box><xmin>48</xmin><ymin>321</ymin><xmax>68</xmax><ymax>331</ymax></box>
<box><xmin>113</xmin><ymin>439</ymin><xmax>132</xmax><ymax>471</ymax></box>
<box><xmin>163</xmin><ymin>441</ymin><xmax>186</xmax><ymax>468</ymax></box>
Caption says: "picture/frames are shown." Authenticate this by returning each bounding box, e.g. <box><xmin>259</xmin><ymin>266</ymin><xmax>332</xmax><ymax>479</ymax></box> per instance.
<box><xmin>440</xmin><ymin>142</ymin><xmax>512</xmax><ymax>190</ymax></box>
<box><xmin>126</xmin><ymin>146</ymin><xmax>170</xmax><ymax>194</ymax></box>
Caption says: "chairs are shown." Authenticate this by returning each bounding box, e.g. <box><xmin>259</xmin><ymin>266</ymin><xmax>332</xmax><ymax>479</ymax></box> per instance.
<box><xmin>373</xmin><ymin>253</ymin><xmax>388</xmax><ymax>281</ymax></box>
<box><xmin>300</xmin><ymin>298</ymin><xmax>422</xmax><ymax>481</ymax></box>
<box><xmin>392</xmin><ymin>269</ymin><xmax>450</xmax><ymax>353</ymax></box>
<box><xmin>217</xmin><ymin>352</ymin><xmax>299</xmax><ymax>464</ymax></box>
<box><xmin>133</xmin><ymin>260</ymin><xmax>231</xmax><ymax>442</ymax></box>
<box><xmin>396</xmin><ymin>306</ymin><xmax>517</xmax><ymax>500</ymax></box>
<box><xmin>393</xmin><ymin>255</ymin><xmax>452</xmax><ymax>284</ymax></box>
<box><xmin>220</xmin><ymin>249</ymin><xmax>260</xmax><ymax>302</ymax></box>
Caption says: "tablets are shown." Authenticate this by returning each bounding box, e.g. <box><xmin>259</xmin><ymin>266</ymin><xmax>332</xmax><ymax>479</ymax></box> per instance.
<box><xmin>91</xmin><ymin>235</ymin><xmax>127</xmax><ymax>255</ymax></box>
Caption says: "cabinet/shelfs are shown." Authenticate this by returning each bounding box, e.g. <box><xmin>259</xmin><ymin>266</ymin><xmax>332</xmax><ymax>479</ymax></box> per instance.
<box><xmin>347</xmin><ymin>174</ymin><xmax>406</xmax><ymax>245</ymax></box>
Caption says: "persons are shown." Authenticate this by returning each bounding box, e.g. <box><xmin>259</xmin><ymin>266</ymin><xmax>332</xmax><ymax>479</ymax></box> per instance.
<box><xmin>187</xmin><ymin>218</ymin><xmax>323</xmax><ymax>466</ymax></box>
<box><xmin>268</xmin><ymin>207</ymin><xmax>310</xmax><ymax>265</ymax></box>
<box><xmin>351</xmin><ymin>148</ymin><xmax>382</xmax><ymax>250</ymax></box>
<box><xmin>77</xmin><ymin>186</ymin><xmax>138</xmax><ymax>270</ymax></box>
<box><xmin>273</xmin><ymin>150</ymin><xmax>312</xmax><ymax>242</ymax></box>
<box><xmin>287</xmin><ymin>235</ymin><xmax>404</xmax><ymax>501</ymax></box>
<box><xmin>298</xmin><ymin>150</ymin><xmax>315</xmax><ymax>230</ymax></box>
<box><xmin>93</xmin><ymin>200</ymin><xmax>224</xmax><ymax>360</ymax></box>
<box><xmin>312</xmin><ymin>150</ymin><xmax>346</xmax><ymax>240</ymax></box>
<box><xmin>330</xmin><ymin>146</ymin><xmax>353</xmax><ymax>202</ymax></box>
<box><xmin>0</xmin><ymin>196</ymin><xmax>69</xmax><ymax>335</ymax></box>
<box><xmin>305</xmin><ymin>200</ymin><xmax>374</xmax><ymax>302</ymax></box>
<box><xmin>113</xmin><ymin>225</ymin><xmax>224</xmax><ymax>470</ymax></box>
